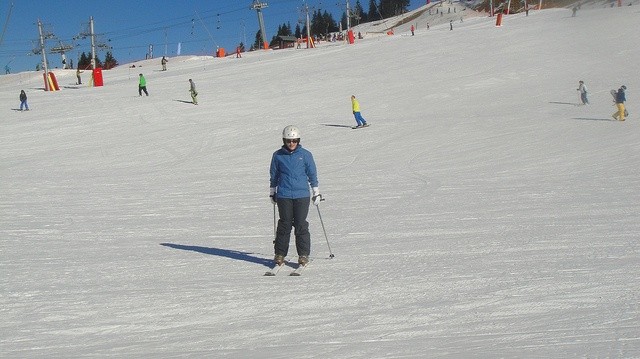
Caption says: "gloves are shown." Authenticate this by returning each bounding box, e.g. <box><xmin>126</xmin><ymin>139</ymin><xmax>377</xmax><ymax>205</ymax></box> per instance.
<box><xmin>269</xmin><ymin>188</ymin><xmax>277</xmax><ymax>205</ymax></box>
<box><xmin>313</xmin><ymin>188</ymin><xmax>320</xmax><ymax>205</ymax></box>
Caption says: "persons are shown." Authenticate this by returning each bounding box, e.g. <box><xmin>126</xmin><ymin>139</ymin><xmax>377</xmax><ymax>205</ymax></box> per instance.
<box><xmin>613</xmin><ymin>85</ymin><xmax>626</xmax><ymax>121</ymax></box>
<box><xmin>295</xmin><ymin>37</ymin><xmax>300</xmax><ymax>49</ymax></box>
<box><xmin>19</xmin><ymin>90</ymin><xmax>28</xmax><ymax>111</ymax></box>
<box><xmin>449</xmin><ymin>19</ymin><xmax>453</xmax><ymax>30</ymax></box>
<box><xmin>161</xmin><ymin>56</ymin><xmax>167</xmax><ymax>70</ymax></box>
<box><xmin>189</xmin><ymin>79</ymin><xmax>198</xmax><ymax>103</ymax></box>
<box><xmin>138</xmin><ymin>74</ymin><xmax>148</xmax><ymax>96</ymax></box>
<box><xmin>270</xmin><ymin>125</ymin><xmax>320</xmax><ymax>266</ymax></box>
<box><xmin>74</xmin><ymin>68</ymin><xmax>84</xmax><ymax>84</ymax></box>
<box><xmin>411</xmin><ymin>25</ymin><xmax>414</xmax><ymax>34</ymax></box>
<box><xmin>577</xmin><ymin>81</ymin><xmax>589</xmax><ymax>104</ymax></box>
<box><xmin>351</xmin><ymin>96</ymin><xmax>367</xmax><ymax>128</ymax></box>
<box><xmin>236</xmin><ymin>47</ymin><xmax>240</xmax><ymax>58</ymax></box>
<box><xmin>70</xmin><ymin>61</ymin><xmax>75</xmax><ymax>70</ymax></box>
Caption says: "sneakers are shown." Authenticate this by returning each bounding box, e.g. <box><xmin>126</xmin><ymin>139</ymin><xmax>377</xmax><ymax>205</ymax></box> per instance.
<box><xmin>619</xmin><ymin>119</ymin><xmax>625</xmax><ymax>121</ymax></box>
<box><xmin>357</xmin><ymin>125</ymin><xmax>361</xmax><ymax>128</ymax></box>
<box><xmin>275</xmin><ymin>254</ymin><xmax>283</xmax><ymax>264</ymax></box>
<box><xmin>612</xmin><ymin>114</ymin><xmax>617</xmax><ymax>120</ymax></box>
<box><xmin>363</xmin><ymin>123</ymin><xmax>367</xmax><ymax>126</ymax></box>
<box><xmin>298</xmin><ymin>255</ymin><xmax>308</xmax><ymax>264</ymax></box>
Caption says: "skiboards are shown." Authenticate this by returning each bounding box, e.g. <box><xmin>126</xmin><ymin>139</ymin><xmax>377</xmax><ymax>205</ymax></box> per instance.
<box><xmin>264</xmin><ymin>253</ymin><xmax>316</xmax><ymax>276</ymax></box>
<box><xmin>352</xmin><ymin>124</ymin><xmax>371</xmax><ymax>129</ymax></box>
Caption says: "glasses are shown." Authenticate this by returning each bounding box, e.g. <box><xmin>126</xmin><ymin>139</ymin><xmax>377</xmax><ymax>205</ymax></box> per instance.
<box><xmin>285</xmin><ymin>139</ymin><xmax>297</xmax><ymax>143</ymax></box>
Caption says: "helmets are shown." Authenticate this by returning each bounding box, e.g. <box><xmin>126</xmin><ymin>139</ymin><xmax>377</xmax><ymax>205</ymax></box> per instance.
<box><xmin>283</xmin><ymin>125</ymin><xmax>299</xmax><ymax>139</ymax></box>
